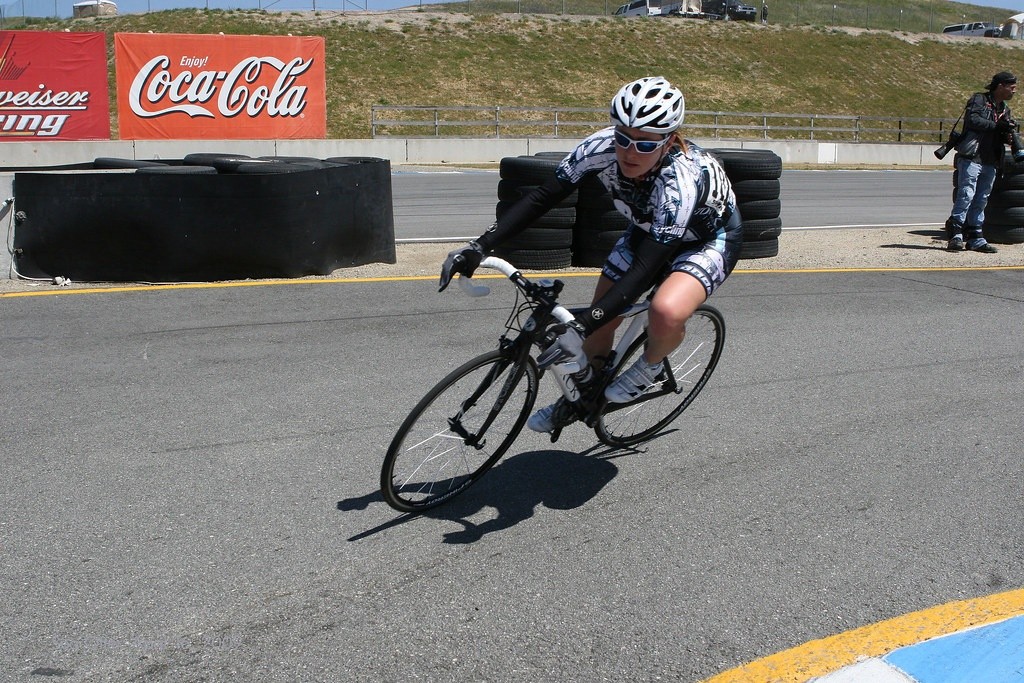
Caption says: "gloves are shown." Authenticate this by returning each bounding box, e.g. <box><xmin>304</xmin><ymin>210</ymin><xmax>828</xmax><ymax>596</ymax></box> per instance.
<box><xmin>537</xmin><ymin>319</ymin><xmax>589</xmax><ymax>368</ymax></box>
<box><xmin>440</xmin><ymin>240</ymin><xmax>484</xmax><ymax>285</ymax></box>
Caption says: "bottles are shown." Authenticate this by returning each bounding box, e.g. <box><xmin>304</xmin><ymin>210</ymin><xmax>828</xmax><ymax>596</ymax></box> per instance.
<box><xmin>556</xmin><ymin>326</ymin><xmax>592</xmax><ymax>384</ymax></box>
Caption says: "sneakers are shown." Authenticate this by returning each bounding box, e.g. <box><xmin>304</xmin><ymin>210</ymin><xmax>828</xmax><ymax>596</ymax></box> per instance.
<box><xmin>965</xmin><ymin>242</ymin><xmax>998</xmax><ymax>252</ymax></box>
<box><xmin>948</xmin><ymin>237</ymin><xmax>963</xmax><ymax>249</ymax></box>
<box><xmin>528</xmin><ymin>402</ymin><xmax>579</xmax><ymax>433</ymax></box>
<box><xmin>604</xmin><ymin>349</ymin><xmax>663</xmax><ymax>403</ymax></box>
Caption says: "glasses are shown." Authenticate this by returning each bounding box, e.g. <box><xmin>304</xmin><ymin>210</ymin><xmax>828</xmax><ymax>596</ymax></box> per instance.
<box><xmin>614</xmin><ymin>124</ymin><xmax>671</xmax><ymax>154</ymax></box>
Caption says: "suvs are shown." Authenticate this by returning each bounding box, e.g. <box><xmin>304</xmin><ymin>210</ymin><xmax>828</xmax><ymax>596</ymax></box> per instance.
<box><xmin>703</xmin><ymin>0</ymin><xmax>757</xmax><ymax>23</ymax></box>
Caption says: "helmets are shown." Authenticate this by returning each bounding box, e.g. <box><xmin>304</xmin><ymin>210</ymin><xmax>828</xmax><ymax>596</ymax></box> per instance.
<box><xmin>610</xmin><ymin>76</ymin><xmax>685</xmax><ymax>134</ymax></box>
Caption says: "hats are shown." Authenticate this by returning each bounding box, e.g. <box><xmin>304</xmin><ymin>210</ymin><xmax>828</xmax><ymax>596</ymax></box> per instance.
<box><xmin>984</xmin><ymin>71</ymin><xmax>1017</xmax><ymax>91</ymax></box>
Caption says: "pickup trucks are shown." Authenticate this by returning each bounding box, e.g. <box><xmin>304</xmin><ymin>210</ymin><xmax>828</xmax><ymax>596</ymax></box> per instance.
<box><xmin>613</xmin><ymin>0</ymin><xmax>681</xmax><ymax>17</ymax></box>
<box><xmin>941</xmin><ymin>21</ymin><xmax>1002</xmax><ymax>37</ymax></box>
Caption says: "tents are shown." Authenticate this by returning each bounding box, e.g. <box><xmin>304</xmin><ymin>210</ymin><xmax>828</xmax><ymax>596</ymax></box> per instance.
<box><xmin>998</xmin><ymin>13</ymin><xmax>1024</xmax><ymax>40</ymax></box>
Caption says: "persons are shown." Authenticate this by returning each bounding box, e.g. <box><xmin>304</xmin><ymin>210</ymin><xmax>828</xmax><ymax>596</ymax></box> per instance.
<box><xmin>760</xmin><ymin>4</ymin><xmax>768</xmax><ymax>22</ymax></box>
<box><xmin>945</xmin><ymin>71</ymin><xmax>1017</xmax><ymax>253</ymax></box>
<box><xmin>438</xmin><ymin>75</ymin><xmax>745</xmax><ymax>432</ymax></box>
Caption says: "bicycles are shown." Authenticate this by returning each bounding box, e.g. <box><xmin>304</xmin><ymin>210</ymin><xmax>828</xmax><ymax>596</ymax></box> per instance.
<box><xmin>381</xmin><ymin>250</ymin><xmax>727</xmax><ymax>515</ymax></box>
